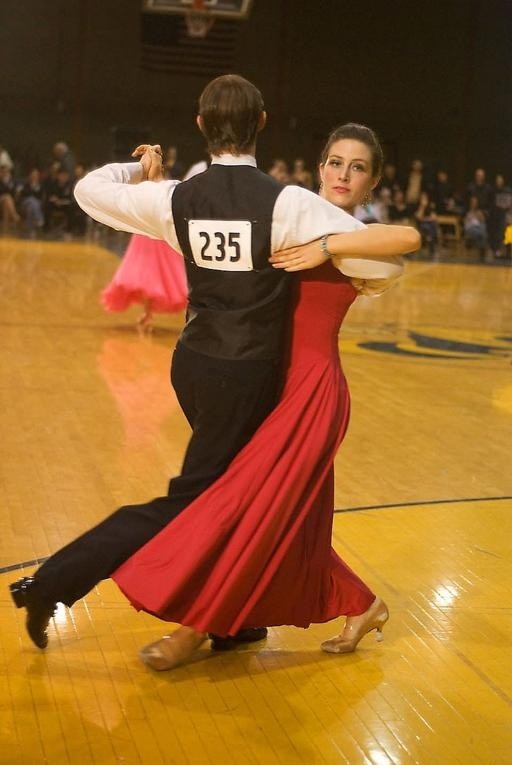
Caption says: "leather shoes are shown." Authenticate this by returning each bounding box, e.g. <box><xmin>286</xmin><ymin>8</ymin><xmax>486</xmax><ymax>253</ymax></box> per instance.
<box><xmin>211</xmin><ymin>628</ymin><xmax>268</xmax><ymax>651</ymax></box>
<box><xmin>10</xmin><ymin>576</ymin><xmax>57</xmax><ymax>647</ymax></box>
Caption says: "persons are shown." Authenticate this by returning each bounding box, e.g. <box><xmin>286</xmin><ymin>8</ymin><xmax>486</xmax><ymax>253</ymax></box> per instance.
<box><xmin>100</xmin><ymin>158</ymin><xmax>188</xmax><ymax>332</ymax></box>
<box><xmin>259</xmin><ymin>150</ymin><xmax>323</xmax><ymax>194</ymax></box>
<box><xmin>353</xmin><ymin>157</ymin><xmax>512</xmax><ymax>263</ymax></box>
<box><xmin>2</xmin><ymin>143</ymin><xmax>91</xmax><ymax>240</ymax></box>
<box><xmin>11</xmin><ymin>74</ymin><xmax>401</xmax><ymax>648</ymax></box>
<box><xmin>112</xmin><ymin>123</ymin><xmax>389</xmax><ymax>671</ymax></box>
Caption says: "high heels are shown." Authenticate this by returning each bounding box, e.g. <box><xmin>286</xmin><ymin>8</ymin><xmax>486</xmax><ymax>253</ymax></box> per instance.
<box><xmin>142</xmin><ymin>635</ymin><xmax>207</xmax><ymax>671</ymax></box>
<box><xmin>320</xmin><ymin>598</ymin><xmax>389</xmax><ymax>654</ymax></box>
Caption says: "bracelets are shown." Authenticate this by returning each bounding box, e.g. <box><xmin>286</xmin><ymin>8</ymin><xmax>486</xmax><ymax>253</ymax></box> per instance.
<box><xmin>321</xmin><ymin>234</ymin><xmax>336</xmax><ymax>256</ymax></box>
